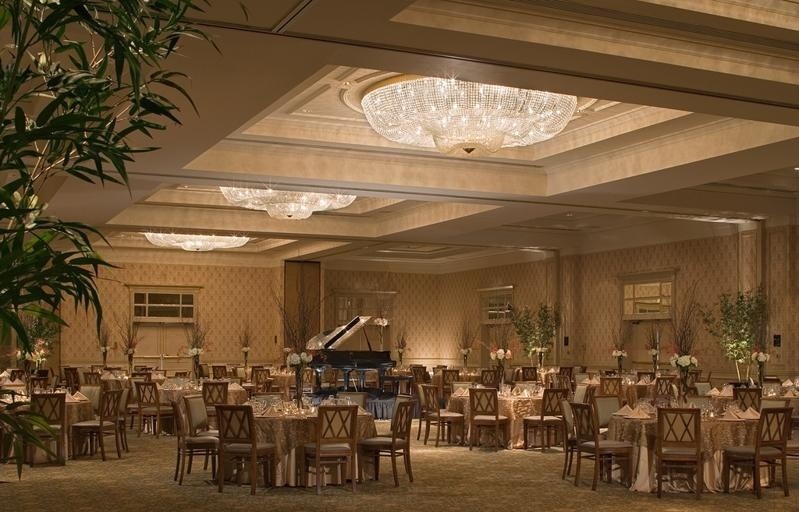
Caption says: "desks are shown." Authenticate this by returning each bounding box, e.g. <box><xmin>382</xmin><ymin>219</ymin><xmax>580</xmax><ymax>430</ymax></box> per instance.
<box><xmin>614</xmin><ymin>409</ymin><xmax>771</xmax><ymax>494</ymax></box>
<box><xmin>218</xmin><ymin>403</ymin><xmax>363</xmax><ymax>488</ymax></box>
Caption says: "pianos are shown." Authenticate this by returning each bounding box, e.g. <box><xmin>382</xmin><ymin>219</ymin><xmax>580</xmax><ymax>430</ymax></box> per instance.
<box><xmin>304</xmin><ymin>316</ymin><xmax>396</xmax><ymax>397</ymax></box>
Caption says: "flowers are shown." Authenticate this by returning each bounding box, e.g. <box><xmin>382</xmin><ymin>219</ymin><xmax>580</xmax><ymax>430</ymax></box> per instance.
<box><xmin>646</xmin><ymin>346</ymin><xmax>660</xmax><ymax>358</ymax></box>
<box><xmin>98</xmin><ymin>346</ymin><xmax>111</xmax><ymax>353</ymax></box>
<box><xmin>608</xmin><ymin>348</ymin><xmax>629</xmax><ymax>358</ymax></box>
<box><xmin>122</xmin><ymin>346</ymin><xmax>136</xmax><ymax>355</ymax></box>
<box><xmin>703</xmin><ymin>292</ymin><xmax>759</xmax><ymax>364</ymax></box>
<box><xmin>240</xmin><ymin>345</ymin><xmax>250</xmax><ymax>353</ymax></box>
<box><xmin>508</xmin><ymin>301</ymin><xmax>560</xmax><ymax>355</ymax></box>
<box><xmin>751</xmin><ymin>347</ymin><xmax>771</xmax><ymax>362</ymax></box>
<box><xmin>670</xmin><ymin>351</ymin><xmax>700</xmax><ymax>369</ymax></box>
<box><xmin>14</xmin><ymin>342</ymin><xmax>49</xmax><ymax>361</ymax></box>
<box><xmin>188</xmin><ymin>347</ymin><xmax>204</xmax><ymax>357</ymax></box>
<box><xmin>284</xmin><ymin>347</ymin><xmax>314</xmax><ymax>362</ymax></box>
<box><xmin>489</xmin><ymin>347</ymin><xmax>514</xmax><ymax>361</ymax></box>
<box><xmin>374</xmin><ymin>317</ymin><xmax>388</xmax><ymax>326</ymax></box>
<box><xmin>396</xmin><ymin>347</ymin><xmax>409</xmax><ymax>353</ymax></box>
<box><xmin>458</xmin><ymin>347</ymin><xmax>472</xmax><ymax>354</ymax></box>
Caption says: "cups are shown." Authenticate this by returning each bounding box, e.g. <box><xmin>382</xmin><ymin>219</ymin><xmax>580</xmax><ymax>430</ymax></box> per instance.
<box><xmin>96</xmin><ymin>367</ymin><xmax>231</xmax><ymax>393</ymax></box>
<box><xmin>597</xmin><ymin>370</ymin><xmax>782</xmax><ymax>421</ymax></box>
<box><xmin>246</xmin><ymin>391</ymin><xmax>353</xmax><ymax>418</ymax></box>
<box><xmin>8</xmin><ymin>385</ymin><xmax>73</xmax><ymax>400</ymax></box>
<box><xmin>395</xmin><ymin>364</ymin><xmax>548</xmax><ymax>400</ymax></box>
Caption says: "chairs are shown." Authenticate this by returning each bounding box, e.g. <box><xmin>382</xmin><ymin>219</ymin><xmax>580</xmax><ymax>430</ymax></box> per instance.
<box><xmin>759</xmin><ymin>395</ymin><xmax>791</xmax><ymax>484</ymax></box>
<box><xmin>723</xmin><ymin>405</ymin><xmax>790</xmax><ymax>497</ymax></box>
<box><xmin>652</xmin><ymin>404</ymin><xmax>706</xmax><ymax>498</ymax></box>
<box><xmin>4</xmin><ymin>364</ymin><xmax>798</xmax><ymax>452</ymax></box>
<box><xmin>171</xmin><ymin>399</ymin><xmax>218</xmax><ymax>484</ymax></box>
<box><xmin>377</xmin><ymin>393</ymin><xmax>413</xmax><ymax>473</ymax></box>
<box><xmin>360</xmin><ymin>401</ymin><xmax>415</xmax><ymax>485</ymax></box>
<box><xmin>182</xmin><ymin>394</ymin><xmax>219</xmax><ymax>473</ymax></box>
<box><xmin>212</xmin><ymin>402</ymin><xmax>275</xmax><ymax>496</ymax></box>
<box><xmin>569</xmin><ymin>400</ymin><xmax>633</xmax><ymax>489</ymax></box>
<box><xmin>72</xmin><ymin>388</ymin><xmax>125</xmax><ymax>461</ymax></box>
<box><xmin>20</xmin><ymin>391</ymin><xmax>67</xmax><ymax>464</ymax></box>
<box><xmin>561</xmin><ymin>384</ymin><xmax>603</xmax><ymax>480</ymax></box>
<box><xmin>301</xmin><ymin>404</ymin><xmax>359</xmax><ymax>493</ymax></box>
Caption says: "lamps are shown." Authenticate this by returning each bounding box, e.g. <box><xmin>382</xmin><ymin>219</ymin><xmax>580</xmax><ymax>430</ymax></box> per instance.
<box><xmin>360</xmin><ymin>74</ymin><xmax>577</xmax><ymax>155</ymax></box>
<box><xmin>219</xmin><ymin>185</ymin><xmax>357</xmax><ymax>220</ymax></box>
<box><xmin>144</xmin><ymin>232</ymin><xmax>250</xmax><ymax>252</ymax></box>
